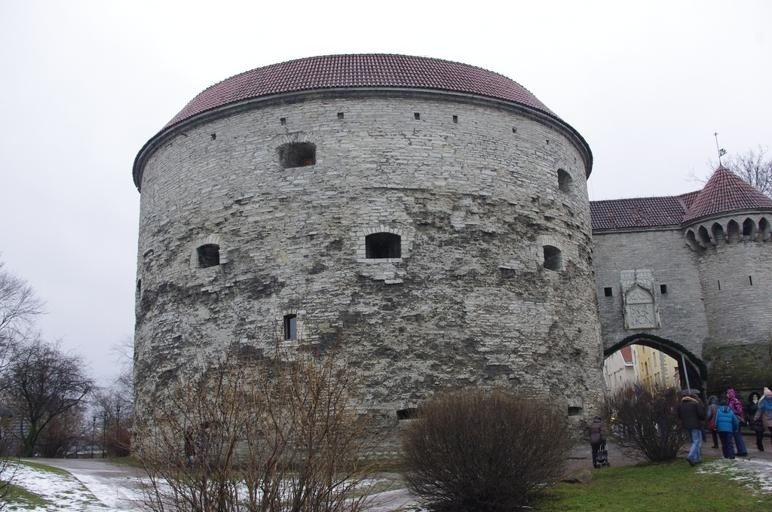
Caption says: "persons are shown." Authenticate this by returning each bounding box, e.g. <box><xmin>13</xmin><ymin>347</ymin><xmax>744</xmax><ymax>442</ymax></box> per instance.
<box><xmin>677</xmin><ymin>384</ymin><xmax>772</xmax><ymax>466</ymax></box>
<box><xmin>588</xmin><ymin>415</ymin><xmax>610</xmax><ymax>469</ymax></box>
<box><xmin>183</xmin><ymin>426</ymin><xmax>197</xmax><ymax>474</ymax></box>
